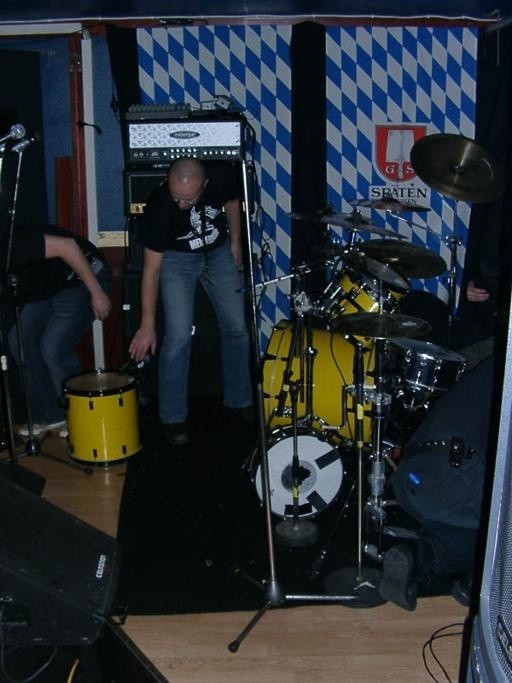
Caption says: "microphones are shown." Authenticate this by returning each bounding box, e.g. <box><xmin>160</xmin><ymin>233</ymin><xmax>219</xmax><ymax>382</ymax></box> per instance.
<box><xmin>12</xmin><ymin>130</ymin><xmax>40</xmax><ymax>152</ymax></box>
<box><xmin>0</xmin><ymin>122</ymin><xmax>25</xmax><ymax>146</ymax></box>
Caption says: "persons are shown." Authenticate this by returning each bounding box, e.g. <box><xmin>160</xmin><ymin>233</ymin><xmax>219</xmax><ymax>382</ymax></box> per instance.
<box><xmin>464</xmin><ymin>265</ymin><xmax>500</xmax><ymax>304</ymax></box>
<box><xmin>379</xmin><ymin>353</ymin><xmax>495</xmax><ymax>611</ymax></box>
<box><xmin>127</xmin><ymin>157</ymin><xmax>257</xmax><ymax>444</ymax></box>
<box><xmin>0</xmin><ymin>198</ymin><xmax>112</xmax><ymax>436</ymax></box>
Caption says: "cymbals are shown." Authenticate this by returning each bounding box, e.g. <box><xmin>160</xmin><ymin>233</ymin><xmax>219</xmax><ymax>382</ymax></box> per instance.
<box><xmin>410</xmin><ymin>132</ymin><xmax>502</xmax><ymax>201</ymax></box>
<box><xmin>334</xmin><ymin>255</ymin><xmax>410</xmax><ymax>313</ymax></box>
<box><xmin>359</xmin><ymin>238</ymin><xmax>446</xmax><ymax>277</ymax></box>
<box><xmin>351</xmin><ymin>198</ymin><xmax>431</xmax><ymax>212</ymax></box>
<box><xmin>288</xmin><ymin>211</ymin><xmax>406</xmax><ymax>237</ymax></box>
<box><xmin>330</xmin><ymin>312</ymin><xmax>431</xmax><ymax>337</ymax></box>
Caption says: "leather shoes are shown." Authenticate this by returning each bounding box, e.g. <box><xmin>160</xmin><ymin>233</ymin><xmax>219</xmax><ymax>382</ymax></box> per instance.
<box><xmin>380</xmin><ymin>543</ymin><xmax>416</xmax><ymax>614</ymax></box>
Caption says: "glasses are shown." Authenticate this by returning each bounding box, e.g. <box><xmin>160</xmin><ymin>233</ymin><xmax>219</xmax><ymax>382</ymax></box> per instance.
<box><xmin>171</xmin><ymin>194</ymin><xmax>201</xmax><ymax>205</ymax></box>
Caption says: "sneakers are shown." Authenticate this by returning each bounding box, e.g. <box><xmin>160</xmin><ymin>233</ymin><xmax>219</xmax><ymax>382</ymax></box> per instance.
<box><xmin>18</xmin><ymin>419</ymin><xmax>67</xmax><ymax>437</ymax></box>
<box><xmin>231</xmin><ymin>402</ymin><xmax>260</xmax><ymax>424</ymax></box>
<box><xmin>163</xmin><ymin>424</ymin><xmax>189</xmax><ymax>445</ymax></box>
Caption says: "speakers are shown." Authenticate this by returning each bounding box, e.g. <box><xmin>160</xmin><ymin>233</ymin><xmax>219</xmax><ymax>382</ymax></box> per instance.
<box><xmin>1</xmin><ymin>476</ymin><xmax>126</xmax><ymax>619</ymax></box>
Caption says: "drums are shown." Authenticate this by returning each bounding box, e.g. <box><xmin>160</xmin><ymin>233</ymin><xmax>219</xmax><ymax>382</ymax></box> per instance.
<box><xmin>249</xmin><ymin>425</ymin><xmax>344</xmax><ymax>520</ymax></box>
<box><xmin>64</xmin><ymin>371</ymin><xmax>144</xmax><ymax>464</ymax></box>
<box><xmin>261</xmin><ymin>318</ymin><xmax>377</xmax><ymax>448</ymax></box>
<box><xmin>377</xmin><ymin>334</ymin><xmax>467</xmax><ymax>414</ymax></box>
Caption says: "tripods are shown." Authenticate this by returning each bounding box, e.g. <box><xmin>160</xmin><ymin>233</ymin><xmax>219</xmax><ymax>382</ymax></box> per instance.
<box><xmin>198</xmin><ymin>161</ymin><xmax>362</xmax><ymax>654</ymax></box>
